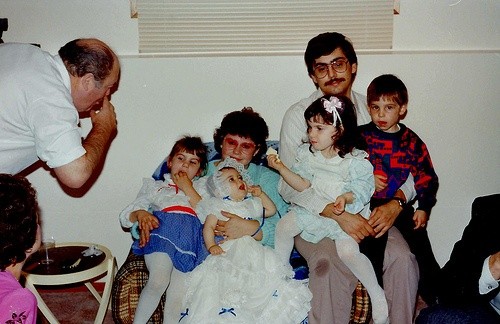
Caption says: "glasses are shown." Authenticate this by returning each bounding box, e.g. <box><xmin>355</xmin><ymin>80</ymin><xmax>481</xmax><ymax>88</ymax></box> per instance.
<box><xmin>223</xmin><ymin>138</ymin><xmax>256</xmax><ymax>154</ymax></box>
<box><xmin>313</xmin><ymin>58</ymin><xmax>349</xmax><ymax>79</ymax></box>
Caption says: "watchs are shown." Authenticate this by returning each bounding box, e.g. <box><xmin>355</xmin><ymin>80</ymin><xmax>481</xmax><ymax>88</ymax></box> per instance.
<box><xmin>392</xmin><ymin>197</ymin><xmax>406</xmax><ymax>208</ymax></box>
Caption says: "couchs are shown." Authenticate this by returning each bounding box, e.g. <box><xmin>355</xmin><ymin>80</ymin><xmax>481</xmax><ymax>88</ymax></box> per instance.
<box><xmin>111</xmin><ymin>141</ymin><xmax>371</xmax><ymax>324</ymax></box>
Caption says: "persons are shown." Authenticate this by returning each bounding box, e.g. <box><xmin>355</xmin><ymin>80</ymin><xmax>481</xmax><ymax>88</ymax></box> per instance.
<box><xmin>118</xmin><ymin>32</ymin><xmax>500</xmax><ymax>324</ymax></box>
<box><xmin>0</xmin><ymin>38</ymin><xmax>118</xmax><ymax>190</ymax></box>
<box><xmin>0</xmin><ymin>172</ymin><xmax>41</xmax><ymax>324</ymax></box>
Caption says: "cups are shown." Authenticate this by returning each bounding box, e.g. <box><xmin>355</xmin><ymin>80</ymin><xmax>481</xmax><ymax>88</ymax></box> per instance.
<box><xmin>38</xmin><ymin>238</ymin><xmax>56</xmax><ymax>265</ymax></box>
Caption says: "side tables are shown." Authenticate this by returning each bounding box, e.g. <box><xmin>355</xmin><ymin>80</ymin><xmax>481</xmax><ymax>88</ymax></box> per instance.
<box><xmin>19</xmin><ymin>242</ymin><xmax>117</xmax><ymax>324</ymax></box>
